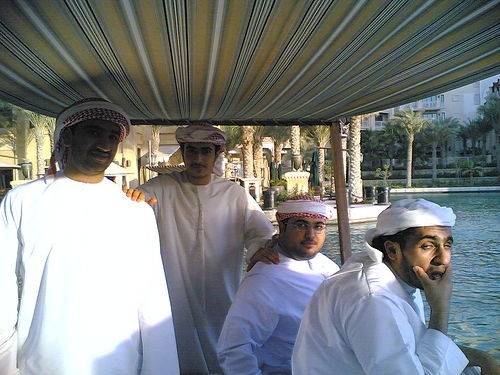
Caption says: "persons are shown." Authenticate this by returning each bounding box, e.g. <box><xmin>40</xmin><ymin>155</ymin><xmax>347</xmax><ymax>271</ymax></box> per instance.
<box><xmin>290</xmin><ymin>196</ymin><xmax>500</xmax><ymax>375</ymax></box>
<box><xmin>213</xmin><ymin>194</ymin><xmax>341</xmax><ymax>374</ymax></box>
<box><xmin>119</xmin><ymin>123</ymin><xmax>280</xmax><ymax>375</ymax></box>
<box><xmin>1</xmin><ymin>98</ymin><xmax>181</xmax><ymax>375</ymax></box>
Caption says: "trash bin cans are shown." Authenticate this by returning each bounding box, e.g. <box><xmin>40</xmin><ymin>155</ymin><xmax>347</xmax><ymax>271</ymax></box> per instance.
<box><xmin>262</xmin><ymin>189</ymin><xmax>276</xmax><ymax>210</ymax></box>
<box><xmin>364</xmin><ymin>185</ymin><xmax>391</xmax><ymax>205</ymax></box>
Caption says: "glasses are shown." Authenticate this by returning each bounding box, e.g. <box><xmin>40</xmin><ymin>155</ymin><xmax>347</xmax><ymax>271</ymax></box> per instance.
<box><xmin>282</xmin><ymin>221</ymin><xmax>327</xmax><ymax>234</ymax></box>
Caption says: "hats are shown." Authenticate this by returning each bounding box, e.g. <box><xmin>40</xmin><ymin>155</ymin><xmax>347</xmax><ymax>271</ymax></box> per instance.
<box><xmin>49</xmin><ymin>97</ymin><xmax>132</xmax><ymax>174</ymax></box>
<box><xmin>276</xmin><ymin>194</ymin><xmax>332</xmax><ymax>221</ymax></box>
<box><xmin>176</xmin><ymin>121</ymin><xmax>227</xmax><ymax>149</ymax></box>
<box><xmin>376</xmin><ymin>199</ymin><xmax>457</xmax><ymax>237</ymax></box>
